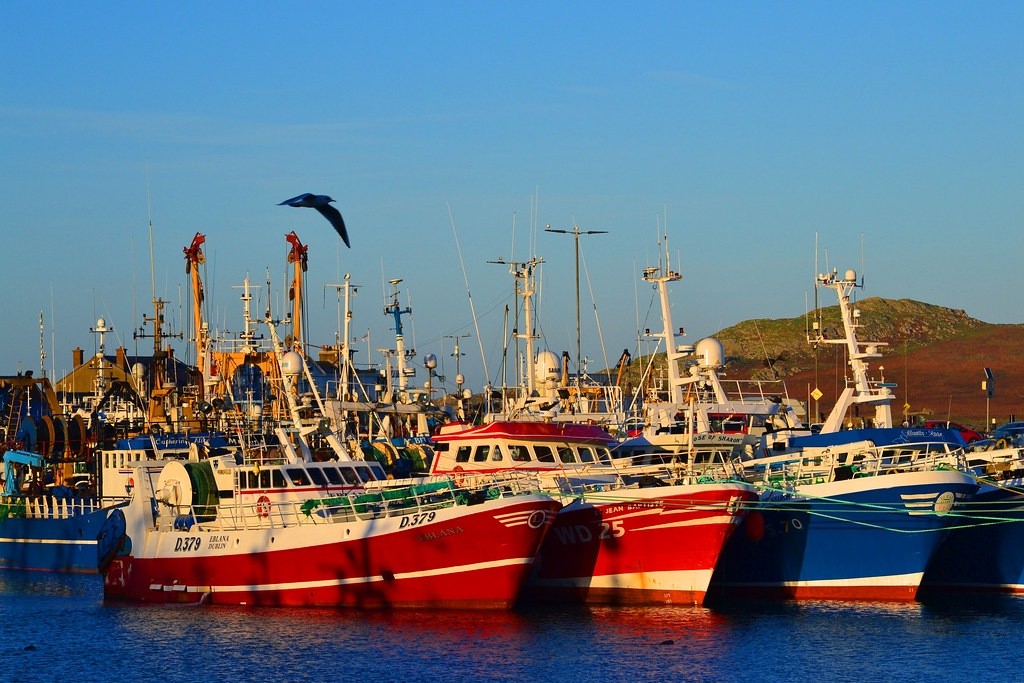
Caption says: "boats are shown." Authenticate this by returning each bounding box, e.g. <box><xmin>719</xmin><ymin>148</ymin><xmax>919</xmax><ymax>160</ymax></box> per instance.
<box><xmin>90</xmin><ymin>426</ymin><xmax>555</xmax><ymax>611</ymax></box>
<box><xmin>0</xmin><ymin>190</ymin><xmax>968</xmax><ymax>578</ymax></box>
<box><xmin>618</xmin><ymin>424</ymin><xmax>972</xmax><ymax>608</ymax></box>
<box><xmin>425</xmin><ymin>397</ymin><xmax>760</xmax><ymax>611</ymax></box>
<box><xmin>940</xmin><ymin>449</ymin><xmax>1021</xmax><ymax>600</ymax></box>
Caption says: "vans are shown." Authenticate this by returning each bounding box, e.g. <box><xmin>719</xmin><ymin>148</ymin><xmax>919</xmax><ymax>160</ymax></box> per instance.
<box><xmin>625</xmin><ymin>412</ymin><xmax>749</xmax><ymax>437</ymax></box>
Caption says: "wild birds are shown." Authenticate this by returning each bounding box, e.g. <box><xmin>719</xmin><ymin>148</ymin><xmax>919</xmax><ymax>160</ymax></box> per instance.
<box><xmin>276</xmin><ymin>193</ymin><xmax>350</xmax><ymax>250</ymax></box>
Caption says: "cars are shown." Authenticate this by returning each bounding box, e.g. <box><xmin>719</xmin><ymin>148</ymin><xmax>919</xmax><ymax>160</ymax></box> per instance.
<box><xmin>989</xmin><ymin>421</ymin><xmax>1024</xmax><ymax>440</ymax></box>
<box><xmin>912</xmin><ymin>418</ymin><xmax>987</xmax><ymax>445</ymax></box>
